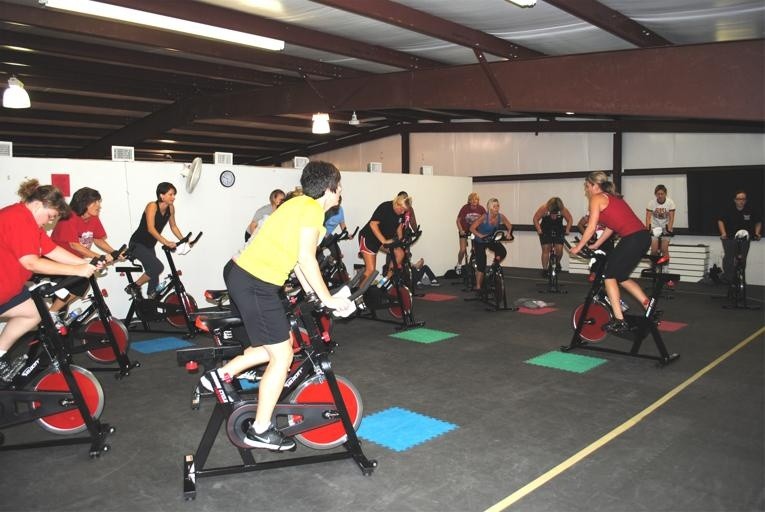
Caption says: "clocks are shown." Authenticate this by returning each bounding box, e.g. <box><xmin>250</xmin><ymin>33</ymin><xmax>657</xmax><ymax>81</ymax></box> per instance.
<box><xmin>220</xmin><ymin>170</ymin><xmax>235</xmax><ymax>187</ymax></box>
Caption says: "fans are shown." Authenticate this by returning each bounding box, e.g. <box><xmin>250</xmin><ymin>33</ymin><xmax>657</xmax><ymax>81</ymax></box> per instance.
<box><xmin>182</xmin><ymin>158</ymin><xmax>202</xmax><ymax>193</ymax></box>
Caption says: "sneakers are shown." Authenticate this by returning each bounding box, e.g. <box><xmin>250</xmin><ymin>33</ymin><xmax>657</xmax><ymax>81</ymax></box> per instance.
<box><xmin>600</xmin><ymin>317</ymin><xmax>630</xmax><ymax>331</ymax></box>
<box><xmin>243</xmin><ymin>422</ymin><xmax>296</xmax><ymax>451</ymax></box>
<box><xmin>456</xmin><ymin>262</ymin><xmax>462</xmax><ymax>276</ymax></box>
<box><xmin>200</xmin><ymin>368</ymin><xmax>241</xmax><ymax>404</ymax></box>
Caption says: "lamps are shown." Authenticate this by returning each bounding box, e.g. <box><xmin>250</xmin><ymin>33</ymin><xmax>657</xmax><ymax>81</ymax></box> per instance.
<box><xmin>112</xmin><ymin>146</ymin><xmax>134</xmax><ymax>162</ymax></box>
<box><xmin>294</xmin><ymin>157</ymin><xmax>309</xmax><ymax>169</ymax></box>
<box><xmin>312</xmin><ymin>112</ymin><xmax>330</xmax><ymax>134</ymax></box>
<box><xmin>0</xmin><ymin>141</ymin><xmax>13</xmax><ymax>156</ymax></box>
<box><xmin>214</xmin><ymin>152</ymin><xmax>233</xmax><ymax>164</ymax></box>
<box><xmin>420</xmin><ymin>166</ymin><xmax>433</xmax><ymax>175</ymax></box>
<box><xmin>349</xmin><ymin>112</ymin><xmax>359</xmax><ymax>126</ymax></box>
<box><xmin>2</xmin><ymin>72</ymin><xmax>31</xmax><ymax>109</ymax></box>
<box><xmin>367</xmin><ymin>162</ymin><xmax>382</xmax><ymax>173</ymax></box>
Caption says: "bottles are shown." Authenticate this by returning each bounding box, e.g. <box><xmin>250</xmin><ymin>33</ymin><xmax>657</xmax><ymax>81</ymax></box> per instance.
<box><xmin>376</xmin><ymin>276</ymin><xmax>389</xmax><ymax>290</ymax></box>
<box><xmin>64</xmin><ymin>306</ymin><xmax>82</xmax><ymax>324</ymax></box>
<box><xmin>1</xmin><ymin>353</ymin><xmax>30</xmax><ymax>384</ymax></box>
<box><xmin>155</xmin><ymin>281</ymin><xmax>166</xmax><ymax>291</ymax></box>
<box><xmin>619</xmin><ymin>299</ymin><xmax>628</xmax><ymax>311</ymax></box>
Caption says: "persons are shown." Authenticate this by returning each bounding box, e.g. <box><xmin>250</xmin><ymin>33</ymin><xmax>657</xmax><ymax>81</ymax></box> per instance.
<box><xmin>455</xmin><ymin>192</ymin><xmax>486</xmax><ymax>274</ymax></box>
<box><xmin>200</xmin><ymin>160</ymin><xmax>360</xmax><ymax>451</ymax></box>
<box><xmin>126</xmin><ymin>183</ymin><xmax>192</xmax><ymax>298</ymax></box>
<box><xmin>570</xmin><ymin>172</ymin><xmax>676</xmax><ymax>334</ymax></box>
<box><xmin>355</xmin><ymin>191</ymin><xmax>443</xmax><ymax>311</ymax></box>
<box><xmin>0</xmin><ymin>177</ymin><xmax>106</xmax><ymax>381</ymax></box>
<box><xmin>717</xmin><ymin>190</ymin><xmax>762</xmax><ymax>282</ymax></box>
<box><xmin>49</xmin><ymin>187</ymin><xmax>125</xmax><ymax>335</ymax></box>
<box><xmin>532</xmin><ymin>198</ymin><xmax>572</xmax><ymax>275</ymax></box>
<box><xmin>469</xmin><ymin>198</ymin><xmax>511</xmax><ymax>291</ymax></box>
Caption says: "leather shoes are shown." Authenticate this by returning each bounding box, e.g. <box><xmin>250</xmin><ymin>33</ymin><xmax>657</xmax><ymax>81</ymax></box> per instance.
<box><xmin>416</xmin><ymin>284</ymin><xmax>429</xmax><ymax>290</ymax></box>
<box><xmin>351</xmin><ymin>287</ymin><xmax>368</xmax><ymax>312</ymax></box>
